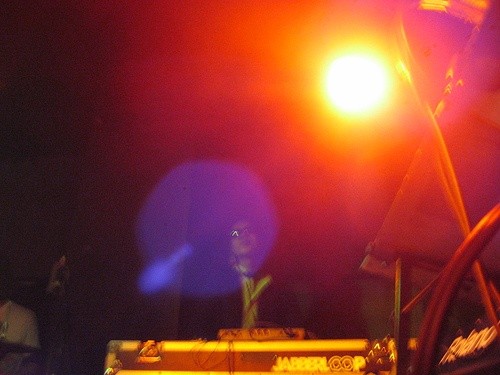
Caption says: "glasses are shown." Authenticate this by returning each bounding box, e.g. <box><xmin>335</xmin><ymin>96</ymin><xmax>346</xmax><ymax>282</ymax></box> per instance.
<box><xmin>230</xmin><ymin>227</ymin><xmax>256</xmax><ymax>237</ymax></box>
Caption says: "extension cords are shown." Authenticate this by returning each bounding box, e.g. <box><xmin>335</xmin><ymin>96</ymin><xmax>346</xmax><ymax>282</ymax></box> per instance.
<box><xmin>217</xmin><ymin>328</ymin><xmax>306</xmax><ymax>341</ymax></box>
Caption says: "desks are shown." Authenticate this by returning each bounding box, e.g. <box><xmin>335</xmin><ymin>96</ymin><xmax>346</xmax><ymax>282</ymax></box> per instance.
<box><xmin>103</xmin><ymin>338</ymin><xmax>368</xmax><ymax>374</ymax></box>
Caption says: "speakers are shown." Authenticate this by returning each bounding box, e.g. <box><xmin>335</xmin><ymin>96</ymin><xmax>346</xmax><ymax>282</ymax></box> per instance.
<box><xmin>101</xmin><ymin>341</ymin><xmax>373</xmax><ymax>375</ymax></box>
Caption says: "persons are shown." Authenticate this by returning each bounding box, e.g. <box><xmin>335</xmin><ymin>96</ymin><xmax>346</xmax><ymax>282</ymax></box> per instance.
<box><xmin>191</xmin><ymin>216</ymin><xmax>316</xmax><ymax>341</ymax></box>
<box><xmin>0</xmin><ymin>247</ymin><xmax>76</xmax><ymax>375</ymax></box>
